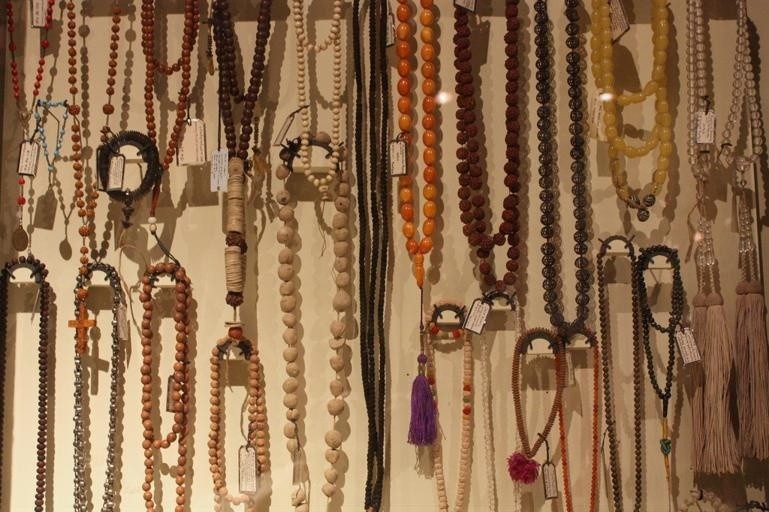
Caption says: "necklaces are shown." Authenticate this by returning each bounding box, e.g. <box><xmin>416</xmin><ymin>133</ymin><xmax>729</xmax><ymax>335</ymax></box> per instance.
<box><xmin>274</xmin><ymin>130</ymin><xmax>353</xmax><ymax>512</ymax></box>
<box><xmin>451</xmin><ymin>0</ymin><xmax>522</xmax><ymax>294</ymax></box>
<box><xmin>5</xmin><ymin>0</ymin><xmax>56</xmax><ymax>253</ymax></box>
<box><xmin>685</xmin><ymin>0</ymin><xmax>748</xmax><ymax>479</ymax></box>
<box><xmin>595</xmin><ymin>233</ymin><xmax>642</xmax><ymax>512</ymax></box>
<box><xmin>72</xmin><ymin>262</ymin><xmax>122</xmax><ymax>511</ymax></box>
<box><xmin>423</xmin><ymin>300</ymin><xmax>475</xmax><ymax>512</ymax></box>
<box><xmin>589</xmin><ymin>0</ymin><xmax>674</xmax><ymax>225</ymax></box>
<box><xmin>139</xmin><ymin>262</ymin><xmax>192</xmax><ymax>512</ymax></box>
<box><xmin>289</xmin><ymin>0</ymin><xmax>343</xmax><ymax>202</ymax></box>
<box><xmin>507</xmin><ymin>326</ymin><xmax>567</xmax><ymax>484</ymax></box>
<box><xmin>395</xmin><ymin>0</ymin><xmax>438</xmax><ymax>447</ymax></box>
<box><xmin>555</xmin><ymin>326</ymin><xmax>600</xmax><ymax>512</ymax></box>
<box><xmin>139</xmin><ymin>0</ymin><xmax>201</xmax><ymax>237</ymax></box>
<box><xmin>478</xmin><ymin>288</ymin><xmax>523</xmax><ymax>512</ymax></box>
<box><xmin>694</xmin><ymin>0</ymin><xmax>769</xmax><ymax>461</ymax></box>
<box><xmin>636</xmin><ymin>244</ymin><xmax>685</xmax><ymax>458</ymax></box>
<box><xmin>207</xmin><ymin>331</ymin><xmax>273</xmax><ymax>512</ymax></box>
<box><xmin>351</xmin><ymin>0</ymin><xmax>390</xmax><ymax>512</ymax></box>
<box><xmin>0</xmin><ymin>255</ymin><xmax>51</xmax><ymax>512</ymax></box>
<box><xmin>209</xmin><ymin>0</ymin><xmax>273</xmax><ymax>343</ymax></box>
<box><xmin>532</xmin><ymin>0</ymin><xmax>590</xmax><ymax>335</ymax></box>
<box><xmin>66</xmin><ymin>0</ymin><xmax>121</xmax><ymax>356</ymax></box>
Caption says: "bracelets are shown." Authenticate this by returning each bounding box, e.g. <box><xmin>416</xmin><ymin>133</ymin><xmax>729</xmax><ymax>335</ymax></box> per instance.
<box><xmin>34</xmin><ymin>100</ymin><xmax>70</xmax><ymax>173</ymax></box>
<box><xmin>98</xmin><ymin>127</ymin><xmax>160</xmax><ymax>231</ymax></box>
